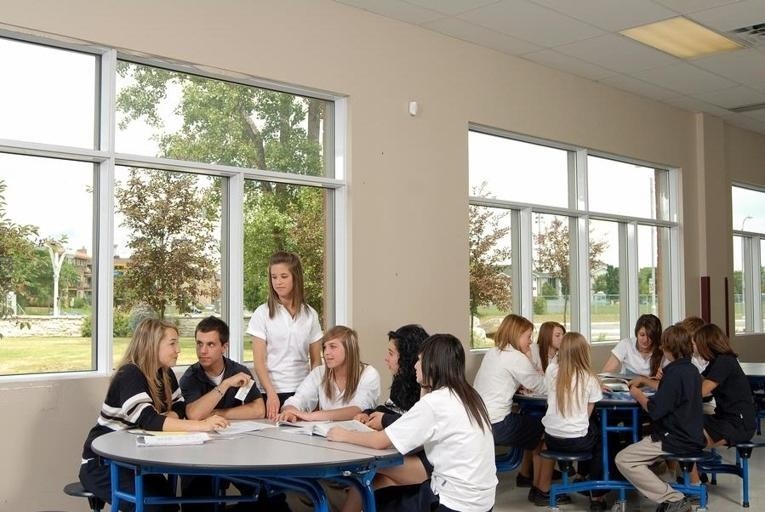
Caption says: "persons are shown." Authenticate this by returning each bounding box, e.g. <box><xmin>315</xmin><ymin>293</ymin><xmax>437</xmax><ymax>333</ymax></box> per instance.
<box><xmin>244</xmin><ymin>251</ymin><xmax>325</xmax><ymax>421</ymax></box>
<box><xmin>615</xmin><ymin>322</ymin><xmax>705</xmax><ymax>511</ymax></box>
<box><xmin>472</xmin><ymin>314</ymin><xmax>553</xmax><ymax>503</ymax></box>
<box><xmin>341</xmin><ymin>323</ymin><xmax>435</xmax><ymax>512</ymax></box>
<box><xmin>178</xmin><ymin>314</ymin><xmax>291</xmax><ymax>512</ymax></box>
<box><xmin>542</xmin><ymin>332</ymin><xmax>612</xmax><ymax>511</ymax></box>
<box><xmin>527</xmin><ymin>321</ymin><xmax>567</xmax><ymax>375</ymax></box>
<box><xmin>602</xmin><ymin>314</ymin><xmax>672</xmax><ymax>381</ymax></box>
<box><xmin>327</xmin><ymin>331</ymin><xmax>501</xmax><ymax>511</ymax></box>
<box><xmin>681</xmin><ymin>316</ymin><xmax>758</xmax><ymax>505</ymax></box>
<box><xmin>77</xmin><ymin>317</ymin><xmax>234</xmax><ymax>512</ymax></box>
<box><xmin>272</xmin><ymin>324</ymin><xmax>382</xmax><ymax>512</ymax></box>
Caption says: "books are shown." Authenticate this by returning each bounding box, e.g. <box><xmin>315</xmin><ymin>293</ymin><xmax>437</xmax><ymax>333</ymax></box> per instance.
<box><xmin>127</xmin><ymin>418</ymin><xmax>380</xmax><ymax>447</ymax></box>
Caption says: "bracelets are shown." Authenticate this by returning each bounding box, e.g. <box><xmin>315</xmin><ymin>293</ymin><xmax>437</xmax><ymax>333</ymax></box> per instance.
<box><xmin>214</xmin><ymin>385</ymin><xmax>224</xmax><ymax>396</ymax></box>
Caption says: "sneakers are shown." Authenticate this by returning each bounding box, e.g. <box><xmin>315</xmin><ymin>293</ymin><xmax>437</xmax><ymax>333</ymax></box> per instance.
<box><xmin>652</xmin><ymin>459</ymin><xmax>709</xmax><ymax>511</ymax></box>
<box><xmin>519</xmin><ymin>466</ymin><xmax>613</xmax><ymax>512</ymax></box>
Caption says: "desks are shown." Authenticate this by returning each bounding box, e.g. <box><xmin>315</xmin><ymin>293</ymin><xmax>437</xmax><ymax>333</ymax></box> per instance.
<box><xmin>511</xmin><ymin>383</ymin><xmax>715</xmax><ymax>512</ymax></box>
<box><xmin>739</xmin><ymin>360</ymin><xmax>765</xmax><ymax>435</ymax></box>
<box><xmin>87</xmin><ymin>418</ymin><xmax>406</xmax><ymax>512</ymax></box>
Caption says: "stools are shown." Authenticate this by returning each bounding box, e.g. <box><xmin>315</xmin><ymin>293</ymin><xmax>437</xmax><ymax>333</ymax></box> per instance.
<box><xmin>722</xmin><ymin>435</ymin><xmax>765</xmax><ymax>508</ymax></box>
<box><xmin>659</xmin><ymin>451</ymin><xmax>712</xmax><ymax>509</ymax></box>
<box><xmin>537</xmin><ymin>447</ymin><xmax>595</xmax><ymax>512</ymax></box>
<box><xmin>63</xmin><ymin>481</ymin><xmax>106</xmax><ymax>511</ymax></box>
